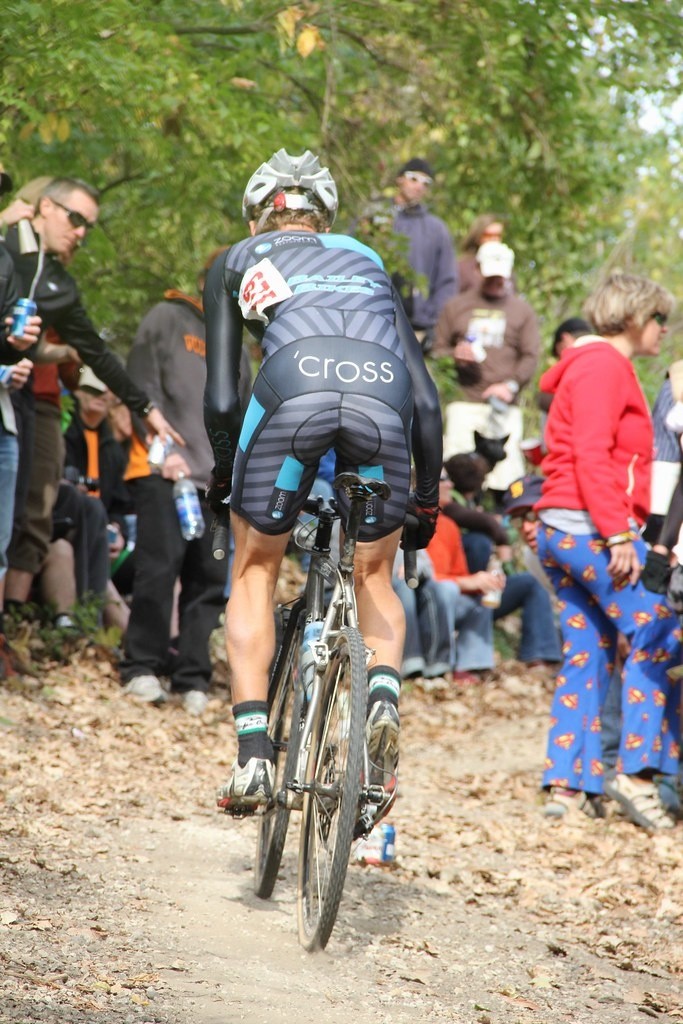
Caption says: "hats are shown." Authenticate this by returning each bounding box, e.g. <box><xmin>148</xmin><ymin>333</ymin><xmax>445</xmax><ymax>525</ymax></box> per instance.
<box><xmin>399</xmin><ymin>157</ymin><xmax>436</xmax><ymax>184</ymax></box>
<box><xmin>502</xmin><ymin>475</ymin><xmax>546</xmax><ymax>513</ymax></box>
<box><xmin>78</xmin><ymin>363</ymin><xmax>109</xmax><ymax>390</ymax></box>
<box><xmin>475</xmin><ymin>240</ymin><xmax>515</xmax><ymax>277</ymax></box>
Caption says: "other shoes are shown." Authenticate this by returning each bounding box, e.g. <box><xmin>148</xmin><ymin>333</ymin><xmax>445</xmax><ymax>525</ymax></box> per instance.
<box><xmin>122</xmin><ymin>675</ymin><xmax>167</xmax><ymax>704</ymax></box>
<box><xmin>415</xmin><ymin>661</ymin><xmax>547</xmax><ymax>694</ymax></box>
<box><xmin>180</xmin><ymin>692</ymin><xmax>209</xmax><ymax>716</ymax></box>
<box><xmin>64</xmin><ymin>626</ymin><xmax>102</xmax><ymax>662</ymax></box>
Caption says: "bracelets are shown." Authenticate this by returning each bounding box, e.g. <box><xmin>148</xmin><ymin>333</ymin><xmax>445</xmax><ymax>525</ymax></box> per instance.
<box><xmin>140</xmin><ymin>401</ymin><xmax>156</xmax><ymax>416</ymax></box>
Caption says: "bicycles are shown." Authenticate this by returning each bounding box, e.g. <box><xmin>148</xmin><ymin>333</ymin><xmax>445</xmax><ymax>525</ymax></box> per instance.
<box><xmin>214</xmin><ymin>472</ymin><xmax>401</xmax><ymax>956</ymax></box>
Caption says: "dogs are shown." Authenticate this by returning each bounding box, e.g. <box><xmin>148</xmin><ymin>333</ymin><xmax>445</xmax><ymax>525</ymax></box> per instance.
<box><xmin>444</xmin><ymin>430</ymin><xmax>511</xmax><ymax>497</ymax></box>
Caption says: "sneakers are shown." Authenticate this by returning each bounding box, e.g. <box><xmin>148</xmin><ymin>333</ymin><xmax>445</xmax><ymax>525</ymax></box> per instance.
<box><xmin>353</xmin><ymin>699</ymin><xmax>400</xmax><ymax>835</ymax></box>
<box><xmin>215</xmin><ymin>757</ymin><xmax>276</xmax><ymax>813</ymax></box>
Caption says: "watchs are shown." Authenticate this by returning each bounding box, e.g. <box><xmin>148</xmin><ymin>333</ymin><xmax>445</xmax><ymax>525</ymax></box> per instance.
<box><xmin>502</xmin><ymin>379</ymin><xmax>520</xmax><ymax>393</ymax></box>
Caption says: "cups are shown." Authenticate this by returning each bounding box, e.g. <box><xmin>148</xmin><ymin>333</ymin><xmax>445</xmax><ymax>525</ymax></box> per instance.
<box><xmin>106</xmin><ymin>524</ymin><xmax>117</xmax><ymax>543</ymax></box>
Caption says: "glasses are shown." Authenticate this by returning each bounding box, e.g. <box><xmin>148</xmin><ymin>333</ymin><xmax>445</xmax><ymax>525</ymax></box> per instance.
<box><xmin>509</xmin><ymin>510</ymin><xmax>538</xmax><ymax>528</ymax></box>
<box><xmin>51</xmin><ymin>198</ymin><xmax>94</xmax><ymax>236</ymax></box>
<box><xmin>650</xmin><ymin>312</ymin><xmax>666</xmax><ymax>324</ymax></box>
<box><xmin>482</xmin><ymin>232</ymin><xmax>505</xmax><ymax>243</ymax></box>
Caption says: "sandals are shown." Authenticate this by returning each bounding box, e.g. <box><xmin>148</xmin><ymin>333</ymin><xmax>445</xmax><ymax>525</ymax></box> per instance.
<box><xmin>603</xmin><ymin>774</ymin><xmax>675</xmax><ymax>833</ymax></box>
<box><xmin>541</xmin><ymin>790</ymin><xmax>599</xmax><ymax>821</ymax></box>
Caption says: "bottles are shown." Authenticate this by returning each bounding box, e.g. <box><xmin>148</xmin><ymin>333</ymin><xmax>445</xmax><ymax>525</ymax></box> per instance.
<box><xmin>380</xmin><ymin>816</ymin><xmax>395</xmax><ymax>861</ymax></box>
<box><xmin>455</xmin><ymin>335</ymin><xmax>480</xmax><ymax>385</ymax></box>
<box><xmin>481</xmin><ymin>560</ymin><xmax>505</xmax><ymax>609</ymax></box>
<box><xmin>300</xmin><ymin>621</ymin><xmax>331</xmax><ymax>701</ymax></box>
<box><xmin>170</xmin><ymin>472</ymin><xmax>205</xmax><ymax>539</ymax></box>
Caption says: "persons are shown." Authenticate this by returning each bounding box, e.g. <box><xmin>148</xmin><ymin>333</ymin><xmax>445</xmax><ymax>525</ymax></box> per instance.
<box><xmin>503</xmin><ymin>271</ymin><xmax>683</xmax><ymax>834</ymax></box>
<box><xmin>204</xmin><ymin>149</ymin><xmax>444</xmax><ymax>833</ymax></box>
<box><xmin>346</xmin><ymin>159</ymin><xmax>541</xmax><ymax>508</ymax></box>
<box><xmin>0</xmin><ymin>173</ymin><xmax>186</xmax><ymax>681</ymax></box>
<box><xmin>320</xmin><ymin>452</ymin><xmax>563</xmax><ymax>698</ymax></box>
<box><xmin>118</xmin><ymin>243</ymin><xmax>253</xmax><ymax>712</ymax></box>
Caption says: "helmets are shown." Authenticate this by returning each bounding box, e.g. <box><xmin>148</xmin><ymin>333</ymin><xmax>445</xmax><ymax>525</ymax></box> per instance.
<box><xmin>242</xmin><ymin>148</ymin><xmax>339</xmax><ymax>227</ymax></box>
